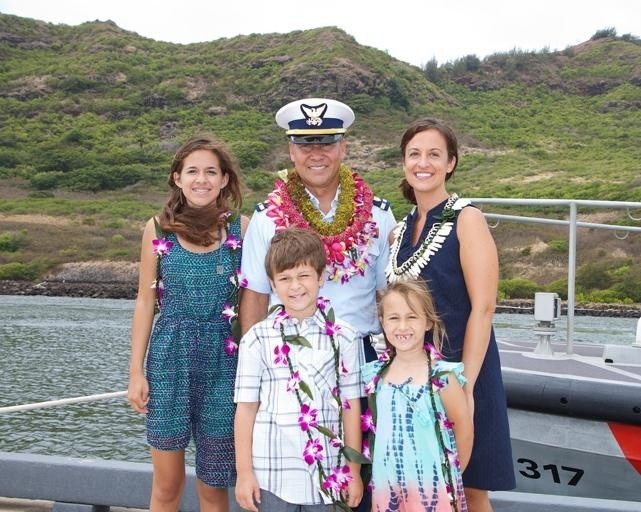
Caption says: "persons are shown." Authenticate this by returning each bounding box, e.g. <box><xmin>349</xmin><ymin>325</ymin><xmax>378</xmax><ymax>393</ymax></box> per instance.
<box><xmin>386</xmin><ymin>116</ymin><xmax>519</xmax><ymax>512</ymax></box>
<box><xmin>232</xmin><ymin>225</ymin><xmax>364</xmax><ymax>512</ymax></box>
<box><xmin>361</xmin><ymin>275</ymin><xmax>476</xmax><ymax>512</ymax></box>
<box><xmin>229</xmin><ymin>97</ymin><xmax>395</xmax><ymax>511</ymax></box>
<box><xmin>127</xmin><ymin>134</ymin><xmax>251</xmax><ymax>512</ymax></box>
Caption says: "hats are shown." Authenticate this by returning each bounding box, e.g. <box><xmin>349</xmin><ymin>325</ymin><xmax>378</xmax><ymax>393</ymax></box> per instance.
<box><xmin>274</xmin><ymin>98</ymin><xmax>355</xmax><ymax>145</ymax></box>
